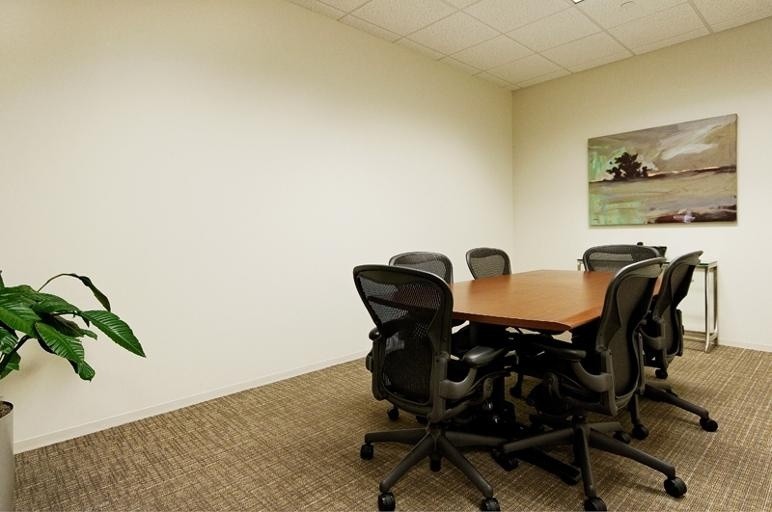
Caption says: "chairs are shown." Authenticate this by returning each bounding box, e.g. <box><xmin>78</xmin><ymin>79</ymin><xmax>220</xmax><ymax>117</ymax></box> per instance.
<box><xmin>354</xmin><ymin>264</ymin><xmax>517</xmax><ymax>512</ymax></box>
<box><xmin>382</xmin><ymin>252</ymin><xmax>512</xmax><ymax>423</ymax></box>
<box><xmin>495</xmin><ymin>258</ymin><xmax>687</xmax><ymax>511</ymax></box>
<box><xmin>575</xmin><ymin>251</ymin><xmax>719</xmax><ymax>433</ymax></box>
<box><xmin>463</xmin><ymin>248</ymin><xmax>566</xmax><ymax>399</ymax></box>
<box><xmin>568</xmin><ymin>246</ymin><xmax>666</xmax><ymax>382</ymax></box>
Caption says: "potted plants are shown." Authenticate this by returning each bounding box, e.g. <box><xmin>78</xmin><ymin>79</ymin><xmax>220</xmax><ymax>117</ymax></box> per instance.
<box><xmin>0</xmin><ymin>268</ymin><xmax>147</xmax><ymax>512</ymax></box>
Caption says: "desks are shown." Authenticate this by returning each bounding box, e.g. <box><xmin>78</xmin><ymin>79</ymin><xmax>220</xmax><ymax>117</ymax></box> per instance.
<box><xmin>368</xmin><ymin>269</ymin><xmax>678</xmax><ymax>332</ymax></box>
